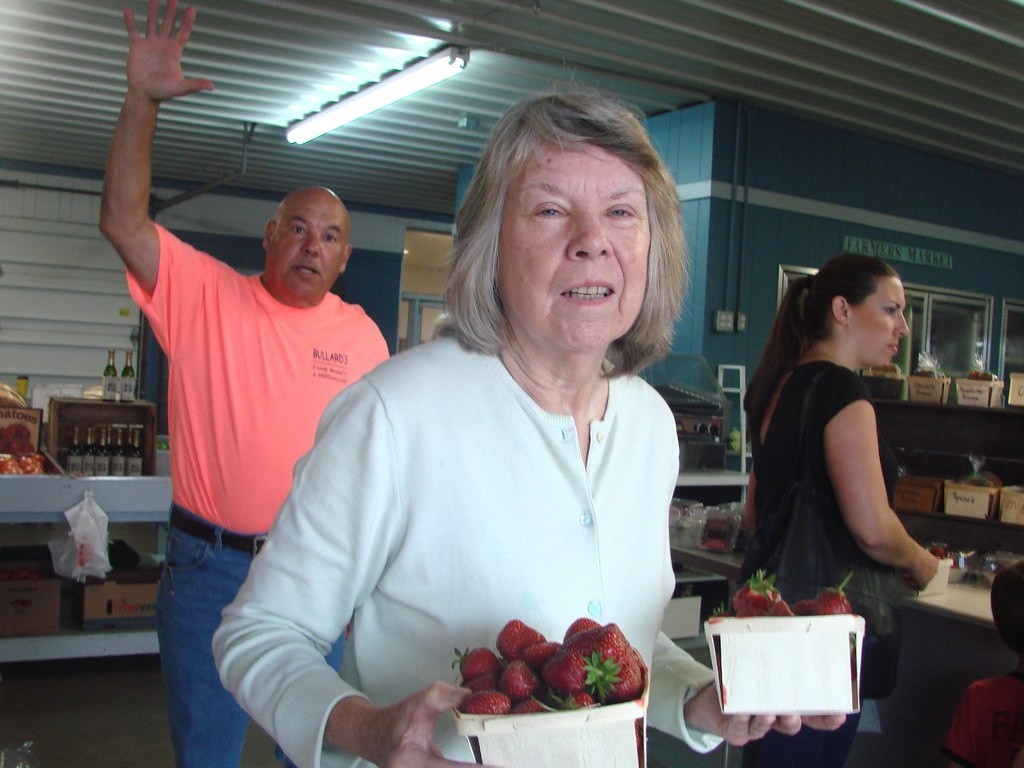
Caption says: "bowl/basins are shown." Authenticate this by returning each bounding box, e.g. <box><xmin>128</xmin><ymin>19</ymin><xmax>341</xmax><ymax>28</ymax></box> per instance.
<box><xmin>948</xmin><ymin>568</ymin><xmax>967</xmax><ymax>582</ymax></box>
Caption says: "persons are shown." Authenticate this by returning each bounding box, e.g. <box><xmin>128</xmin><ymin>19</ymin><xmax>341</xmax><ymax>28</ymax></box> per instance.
<box><xmin>96</xmin><ymin>0</ymin><xmax>392</xmax><ymax>768</ymax></box>
<box><xmin>208</xmin><ymin>91</ymin><xmax>847</xmax><ymax>768</ymax></box>
<box><xmin>741</xmin><ymin>254</ymin><xmax>941</xmax><ymax>767</ymax></box>
<box><xmin>939</xmin><ymin>560</ymin><xmax>1024</xmax><ymax>768</ymax></box>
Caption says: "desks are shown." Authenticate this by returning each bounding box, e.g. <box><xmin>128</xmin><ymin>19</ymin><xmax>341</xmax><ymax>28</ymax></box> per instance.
<box><xmin>48</xmin><ymin>396</ymin><xmax>155</xmax><ymax>476</ymax></box>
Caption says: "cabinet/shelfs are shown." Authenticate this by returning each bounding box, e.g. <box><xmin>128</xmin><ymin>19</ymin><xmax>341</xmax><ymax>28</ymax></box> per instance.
<box><xmin>718</xmin><ymin>365</ymin><xmax>753</xmax><ymax>506</ymax></box>
<box><xmin>862</xmin><ymin>396</ymin><xmax>1023</xmax><ymax>768</ymax></box>
<box><xmin>0</xmin><ymin>472</ymin><xmax>176</xmax><ymax>662</ymax></box>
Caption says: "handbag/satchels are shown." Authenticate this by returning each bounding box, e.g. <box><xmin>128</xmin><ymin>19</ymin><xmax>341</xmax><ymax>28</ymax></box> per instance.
<box><xmin>740</xmin><ymin>367</ymin><xmax>905</xmax><ymax>699</ymax></box>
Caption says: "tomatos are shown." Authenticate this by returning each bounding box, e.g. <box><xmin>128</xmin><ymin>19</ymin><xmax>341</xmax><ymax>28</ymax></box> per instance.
<box><xmin>0</xmin><ymin>453</ymin><xmax>46</xmax><ymax>475</ymax></box>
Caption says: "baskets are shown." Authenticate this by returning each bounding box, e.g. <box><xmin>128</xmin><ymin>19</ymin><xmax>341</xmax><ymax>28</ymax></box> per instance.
<box><xmin>1008</xmin><ymin>372</ymin><xmax>1024</xmax><ymax>406</ymax></box>
<box><xmin>451</xmin><ymin>648</ymin><xmax>649</xmax><ymax>768</ymax></box>
<box><xmin>860</xmin><ymin>368</ymin><xmax>909</xmax><ymax>401</ymax></box>
<box><xmin>909</xmin><ymin>377</ymin><xmax>952</xmax><ymax>406</ymax></box>
<box><xmin>701</xmin><ymin>614</ymin><xmax>868</xmax><ymax>718</ymax></box>
<box><xmin>894</xmin><ymin>475</ymin><xmax>945</xmax><ymax>515</ymax></box>
<box><xmin>943</xmin><ymin>479</ymin><xmax>1001</xmax><ymax>520</ymax></box>
<box><xmin>1000</xmin><ymin>485</ymin><xmax>1024</xmax><ymax>526</ymax></box>
<box><xmin>955</xmin><ymin>377</ymin><xmax>1005</xmax><ymax>408</ymax></box>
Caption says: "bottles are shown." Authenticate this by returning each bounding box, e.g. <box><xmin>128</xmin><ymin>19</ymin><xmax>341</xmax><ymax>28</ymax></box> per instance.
<box><xmin>103</xmin><ymin>350</ymin><xmax>117</xmax><ymax>400</ymax></box>
<box><xmin>120</xmin><ymin>351</ymin><xmax>136</xmax><ymax>401</ymax></box>
<box><xmin>96</xmin><ymin>429</ymin><xmax>113</xmax><ymax>476</ymax></box>
<box><xmin>113</xmin><ymin>427</ymin><xmax>142</xmax><ymax>476</ymax></box>
<box><xmin>67</xmin><ymin>427</ymin><xmax>82</xmax><ymax>475</ymax></box>
<box><xmin>17</xmin><ymin>377</ymin><xmax>28</xmax><ymax>398</ymax></box>
<box><xmin>729</xmin><ymin>427</ymin><xmax>741</xmax><ymax>450</ymax></box>
<box><xmin>82</xmin><ymin>428</ymin><xmax>96</xmax><ymax>475</ymax></box>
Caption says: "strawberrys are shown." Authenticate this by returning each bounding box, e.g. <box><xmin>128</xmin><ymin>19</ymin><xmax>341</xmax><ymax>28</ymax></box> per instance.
<box><xmin>449</xmin><ymin>618</ymin><xmax>643</xmax><ymax>716</ymax></box>
<box><xmin>712</xmin><ymin>567</ymin><xmax>853</xmax><ymax>618</ymax></box>
<box><xmin>927</xmin><ymin>547</ymin><xmax>967</xmax><ymax>569</ymax></box>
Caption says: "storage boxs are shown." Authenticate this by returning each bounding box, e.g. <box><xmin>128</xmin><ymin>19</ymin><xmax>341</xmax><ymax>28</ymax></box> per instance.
<box><xmin>946</xmin><ymin>478</ymin><xmax>999</xmax><ymax>518</ymax></box>
<box><xmin>863</xmin><ymin>559</ymin><xmax>954</xmax><ymax>597</ymax></box>
<box><xmin>0</xmin><ymin>566</ymin><xmax>61</xmax><ymax>636</ymax></box>
<box><xmin>1008</xmin><ymin>372</ymin><xmax>1024</xmax><ymax>406</ymax></box>
<box><xmin>906</xmin><ymin>375</ymin><xmax>951</xmax><ymax>405</ymax></box>
<box><xmin>450</xmin><ymin>649</ymin><xmax>651</xmax><ymax>768</ymax></box>
<box><xmin>76</xmin><ymin>560</ymin><xmax>164</xmax><ymax>631</ymax></box>
<box><xmin>955</xmin><ymin>378</ymin><xmax>1004</xmax><ymax>409</ymax></box>
<box><xmin>999</xmin><ymin>486</ymin><xmax>1024</xmax><ymax>523</ymax></box>
<box><xmin>704</xmin><ymin>614</ymin><xmax>866</xmax><ymax>716</ymax></box>
<box><xmin>893</xmin><ymin>473</ymin><xmax>942</xmax><ymax>512</ymax></box>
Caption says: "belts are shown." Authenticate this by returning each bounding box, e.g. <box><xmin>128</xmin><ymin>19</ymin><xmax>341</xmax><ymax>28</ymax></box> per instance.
<box><xmin>172</xmin><ymin>509</ymin><xmax>268</xmax><ymax>556</ymax></box>
